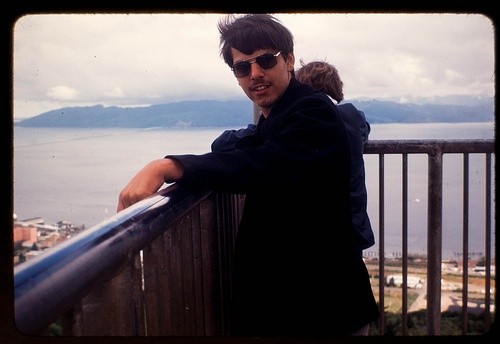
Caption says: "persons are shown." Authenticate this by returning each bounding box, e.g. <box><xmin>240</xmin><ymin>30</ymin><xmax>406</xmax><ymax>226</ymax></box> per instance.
<box><xmin>295</xmin><ymin>58</ymin><xmax>375</xmax><ymax>336</ymax></box>
<box><xmin>116</xmin><ymin>14</ymin><xmax>352</xmax><ymax>337</ymax></box>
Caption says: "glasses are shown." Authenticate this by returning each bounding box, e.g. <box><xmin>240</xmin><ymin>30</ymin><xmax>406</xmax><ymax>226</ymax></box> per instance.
<box><xmin>231</xmin><ymin>50</ymin><xmax>283</xmax><ymax>78</ymax></box>
